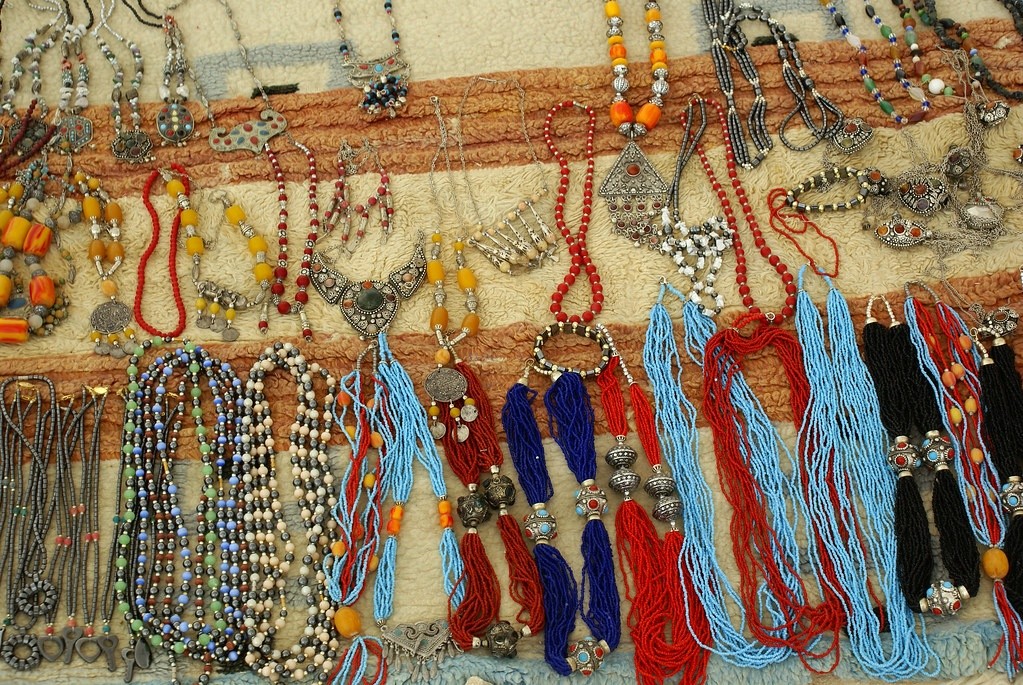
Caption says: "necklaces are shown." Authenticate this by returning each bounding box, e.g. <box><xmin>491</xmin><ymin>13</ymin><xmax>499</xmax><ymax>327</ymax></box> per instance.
<box><xmin>0</xmin><ymin>0</ymin><xmax>1023</xmax><ymax>685</ymax></box>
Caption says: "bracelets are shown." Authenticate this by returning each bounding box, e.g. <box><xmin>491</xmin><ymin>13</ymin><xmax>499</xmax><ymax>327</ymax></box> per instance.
<box><xmin>533</xmin><ymin>322</ymin><xmax>611</xmax><ymax>380</ymax></box>
<box><xmin>786</xmin><ymin>166</ymin><xmax>870</xmax><ymax>213</ymax></box>
